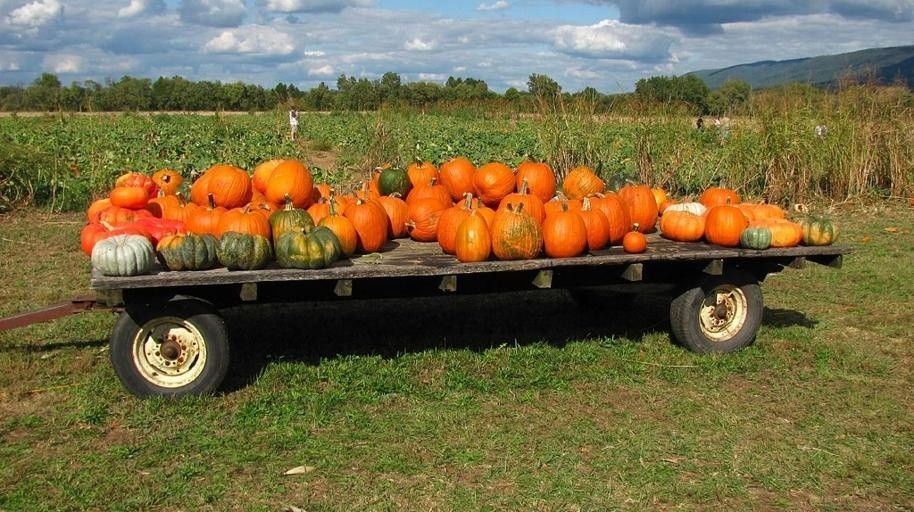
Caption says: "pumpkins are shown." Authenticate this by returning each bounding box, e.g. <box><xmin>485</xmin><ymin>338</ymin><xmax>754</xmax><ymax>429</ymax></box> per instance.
<box><xmin>79</xmin><ymin>155</ymin><xmax>841</xmax><ymax>277</ymax></box>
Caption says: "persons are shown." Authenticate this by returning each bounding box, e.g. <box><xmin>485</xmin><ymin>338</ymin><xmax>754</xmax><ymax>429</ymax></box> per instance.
<box><xmin>693</xmin><ymin>113</ymin><xmax>730</xmax><ymax>130</ymax></box>
<box><xmin>289</xmin><ymin>106</ymin><xmax>301</xmax><ymax>142</ymax></box>
<box><xmin>814</xmin><ymin>122</ymin><xmax>827</xmax><ymax>137</ymax></box>
